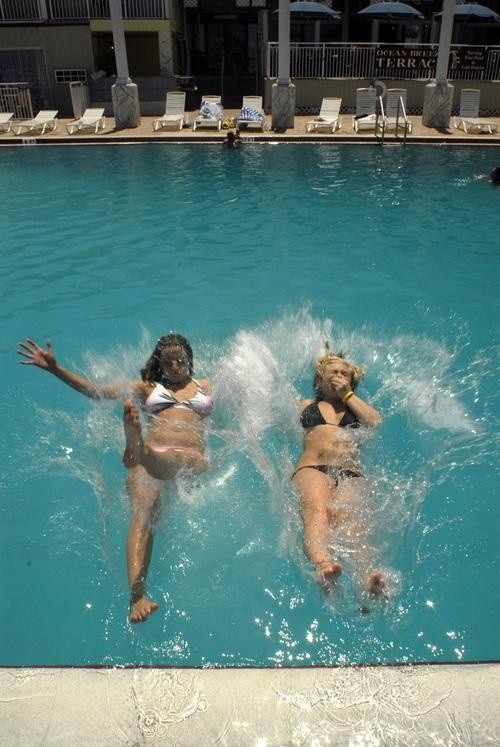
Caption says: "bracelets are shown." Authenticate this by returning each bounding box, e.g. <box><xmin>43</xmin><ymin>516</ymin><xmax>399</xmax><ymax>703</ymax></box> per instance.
<box><xmin>342</xmin><ymin>390</ymin><xmax>354</xmax><ymax>402</ymax></box>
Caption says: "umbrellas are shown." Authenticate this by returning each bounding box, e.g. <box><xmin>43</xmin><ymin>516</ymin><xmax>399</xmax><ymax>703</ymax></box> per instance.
<box><xmin>271</xmin><ymin>0</ymin><xmax>343</xmax><ymax>43</ymax></box>
<box><xmin>356</xmin><ymin>1</ymin><xmax>425</xmax><ymax>42</ymax></box>
<box><xmin>431</xmin><ymin>2</ymin><xmax>500</xmax><ymax>44</ymax></box>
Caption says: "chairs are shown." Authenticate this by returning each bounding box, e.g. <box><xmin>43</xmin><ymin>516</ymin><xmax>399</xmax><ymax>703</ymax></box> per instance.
<box><xmin>151</xmin><ymin>91</ymin><xmax>263</xmax><ymax>133</ymax></box>
<box><xmin>1</xmin><ymin>106</ymin><xmax>106</xmax><ymax>135</ymax></box>
<box><xmin>453</xmin><ymin>88</ymin><xmax>497</xmax><ymax>134</ymax></box>
<box><xmin>352</xmin><ymin>87</ymin><xmax>413</xmax><ymax>133</ymax></box>
<box><xmin>306</xmin><ymin>97</ymin><xmax>343</xmax><ymax>133</ymax></box>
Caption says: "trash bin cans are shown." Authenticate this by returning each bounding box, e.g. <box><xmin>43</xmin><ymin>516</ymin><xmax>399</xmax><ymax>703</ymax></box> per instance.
<box><xmin>180</xmin><ymin>76</ymin><xmax>198</xmax><ymax>111</ymax></box>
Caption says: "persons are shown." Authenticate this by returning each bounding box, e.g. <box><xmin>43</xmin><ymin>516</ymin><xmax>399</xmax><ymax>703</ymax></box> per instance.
<box><xmin>16</xmin><ymin>332</ymin><xmax>214</xmax><ymax>626</ymax></box>
<box><xmin>489</xmin><ymin>165</ymin><xmax>499</xmax><ymax>186</ymax></box>
<box><xmin>287</xmin><ymin>352</ymin><xmax>387</xmax><ymax>616</ymax></box>
<box><xmin>223</xmin><ymin>129</ymin><xmax>242</xmax><ymax>146</ymax></box>
